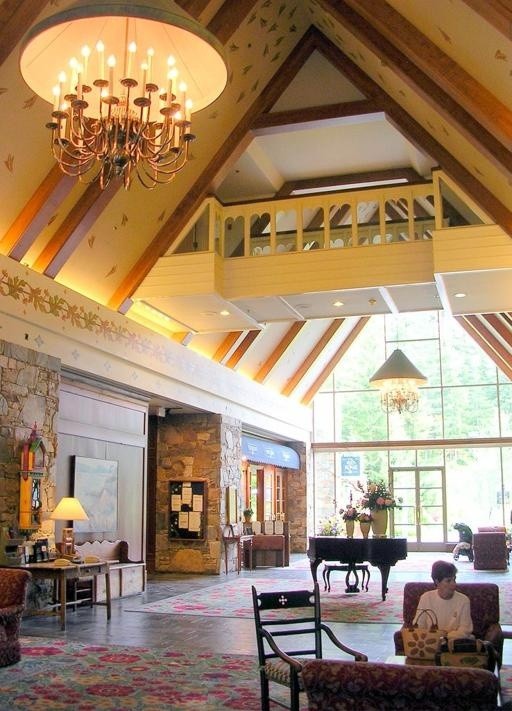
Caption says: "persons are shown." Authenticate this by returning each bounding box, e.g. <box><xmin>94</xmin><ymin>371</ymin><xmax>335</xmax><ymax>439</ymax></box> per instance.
<box><xmin>412</xmin><ymin>561</ymin><xmax>475</xmax><ymax>639</ymax></box>
<box><xmin>452</xmin><ymin>523</ymin><xmax>473</xmax><ymax>561</ymax></box>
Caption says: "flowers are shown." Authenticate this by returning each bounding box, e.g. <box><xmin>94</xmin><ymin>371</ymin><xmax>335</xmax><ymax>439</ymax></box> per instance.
<box><xmin>354</xmin><ymin>512</ymin><xmax>372</xmax><ymax>522</ymax></box>
<box><xmin>356</xmin><ymin>478</ymin><xmax>403</xmax><ymax>513</ymax></box>
<box><xmin>339</xmin><ymin>504</ymin><xmax>359</xmax><ymax>520</ymax></box>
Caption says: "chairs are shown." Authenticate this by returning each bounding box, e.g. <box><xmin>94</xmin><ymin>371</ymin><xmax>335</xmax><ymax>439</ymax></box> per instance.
<box><xmin>248</xmin><ymin>580</ymin><xmax>369</xmax><ymax>711</ymax></box>
<box><xmin>302</xmin><ymin>654</ymin><xmax>502</xmax><ymax>710</ymax></box>
<box><xmin>219</xmin><ymin>520</ymin><xmax>255</xmax><ymax>577</ymax></box>
<box><xmin>477</xmin><ymin>525</ymin><xmax>512</xmax><ymax>567</ymax></box>
<box><xmin>394</xmin><ymin>580</ymin><xmax>505</xmax><ymax>668</ymax></box>
<box><xmin>472</xmin><ymin>532</ymin><xmax>509</xmax><ymax>571</ymax></box>
<box><xmin>0</xmin><ymin>566</ymin><xmax>34</xmax><ymax>669</ymax></box>
<box><xmin>451</xmin><ymin>521</ymin><xmax>474</xmax><ymax>563</ymax></box>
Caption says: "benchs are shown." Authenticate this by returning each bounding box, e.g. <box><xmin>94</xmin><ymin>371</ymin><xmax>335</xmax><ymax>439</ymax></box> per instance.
<box><xmin>69</xmin><ymin>537</ymin><xmax>151</xmax><ymax>606</ymax></box>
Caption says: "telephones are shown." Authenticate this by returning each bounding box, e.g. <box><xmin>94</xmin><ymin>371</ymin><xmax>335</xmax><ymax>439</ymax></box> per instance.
<box><xmin>54</xmin><ymin>559</ymin><xmax>71</xmax><ymax>566</ymax></box>
<box><xmin>85</xmin><ymin>555</ymin><xmax>100</xmax><ymax>563</ymax></box>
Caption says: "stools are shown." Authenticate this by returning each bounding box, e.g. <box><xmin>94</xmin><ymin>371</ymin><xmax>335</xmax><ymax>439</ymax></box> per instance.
<box><xmin>321</xmin><ymin>562</ymin><xmax>373</xmax><ymax>594</ymax></box>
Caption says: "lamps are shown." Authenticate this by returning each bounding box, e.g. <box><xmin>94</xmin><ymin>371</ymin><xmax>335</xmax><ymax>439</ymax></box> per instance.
<box><xmin>365</xmin><ymin>347</ymin><xmax>429</xmax><ymax>412</ymax></box>
<box><xmin>49</xmin><ymin>496</ymin><xmax>89</xmax><ymax>563</ymax></box>
<box><xmin>16</xmin><ymin>0</ymin><xmax>234</xmax><ymax>192</ymax></box>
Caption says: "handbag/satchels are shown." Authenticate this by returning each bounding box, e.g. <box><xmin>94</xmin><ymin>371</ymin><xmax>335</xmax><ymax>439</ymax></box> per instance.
<box><xmin>402</xmin><ymin>626</ymin><xmax>447</xmax><ymax>661</ymax></box>
<box><xmin>435</xmin><ymin>637</ymin><xmax>496</xmax><ymax>671</ymax></box>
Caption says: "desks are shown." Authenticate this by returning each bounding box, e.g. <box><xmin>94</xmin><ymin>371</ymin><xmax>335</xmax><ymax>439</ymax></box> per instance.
<box><xmin>4</xmin><ymin>556</ymin><xmax>121</xmax><ymax>633</ymax></box>
<box><xmin>377</xmin><ymin>653</ymin><xmax>488</xmax><ymax>670</ymax></box>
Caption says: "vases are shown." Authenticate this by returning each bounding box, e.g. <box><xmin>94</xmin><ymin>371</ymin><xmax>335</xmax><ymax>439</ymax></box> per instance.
<box><xmin>360</xmin><ymin>520</ymin><xmax>371</xmax><ymax>541</ymax></box>
<box><xmin>344</xmin><ymin>518</ymin><xmax>355</xmax><ymax>539</ymax></box>
<box><xmin>369</xmin><ymin>507</ymin><xmax>390</xmax><ymax>541</ymax></box>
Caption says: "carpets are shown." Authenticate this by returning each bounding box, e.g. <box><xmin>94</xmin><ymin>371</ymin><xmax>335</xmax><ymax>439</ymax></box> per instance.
<box><xmin>123</xmin><ymin>576</ymin><xmax>512</xmax><ymax>627</ymax></box>
<box><xmin>266</xmin><ymin>548</ymin><xmax>512</xmax><ymax>572</ymax></box>
<box><xmin>1</xmin><ymin>632</ymin><xmax>511</xmax><ymax>711</ymax></box>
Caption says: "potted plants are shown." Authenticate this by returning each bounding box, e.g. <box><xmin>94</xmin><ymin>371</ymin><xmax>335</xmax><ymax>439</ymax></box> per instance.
<box><xmin>241</xmin><ymin>509</ymin><xmax>254</xmax><ymax>522</ymax></box>
